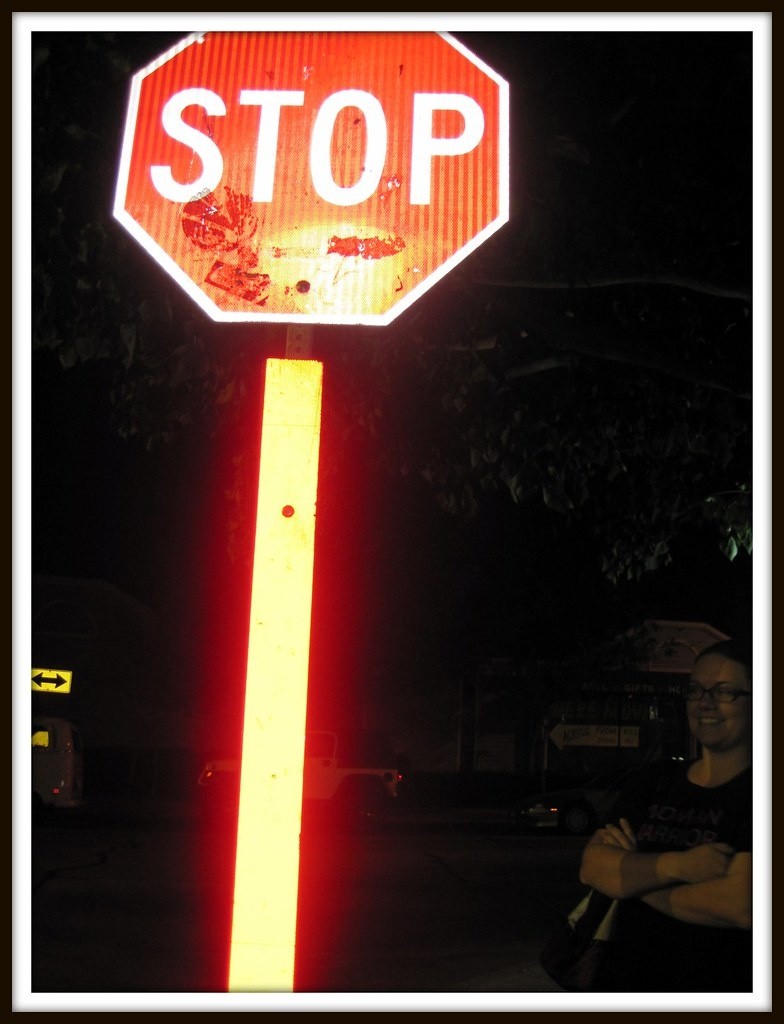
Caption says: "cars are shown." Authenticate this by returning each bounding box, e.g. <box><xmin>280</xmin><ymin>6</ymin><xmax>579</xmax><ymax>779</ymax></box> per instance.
<box><xmin>514</xmin><ymin>766</ymin><xmax>641</xmax><ymax>837</ymax></box>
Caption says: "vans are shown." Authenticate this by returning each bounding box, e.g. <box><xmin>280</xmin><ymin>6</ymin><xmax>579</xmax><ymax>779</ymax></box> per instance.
<box><xmin>32</xmin><ymin>715</ymin><xmax>87</xmax><ymax>826</ymax></box>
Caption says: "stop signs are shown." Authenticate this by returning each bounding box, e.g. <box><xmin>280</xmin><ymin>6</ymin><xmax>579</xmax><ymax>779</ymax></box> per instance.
<box><xmin>112</xmin><ymin>32</ymin><xmax>510</xmax><ymax>328</ymax></box>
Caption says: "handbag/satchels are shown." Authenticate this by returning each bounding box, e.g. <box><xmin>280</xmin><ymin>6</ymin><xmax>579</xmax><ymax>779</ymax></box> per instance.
<box><xmin>539</xmin><ymin>758</ymin><xmax>660</xmax><ymax>991</ymax></box>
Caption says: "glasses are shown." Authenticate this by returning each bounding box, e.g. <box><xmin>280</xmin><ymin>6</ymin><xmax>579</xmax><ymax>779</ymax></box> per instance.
<box><xmin>681</xmin><ymin>681</ymin><xmax>751</xmax><ymax>703</ymax></box>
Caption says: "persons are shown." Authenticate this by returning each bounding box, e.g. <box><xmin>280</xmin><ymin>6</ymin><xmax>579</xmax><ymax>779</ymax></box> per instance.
<box><xmin>579</xmin><ymin>639</ymin><xmax>752</xmax><ymax>993</ymax></box>
